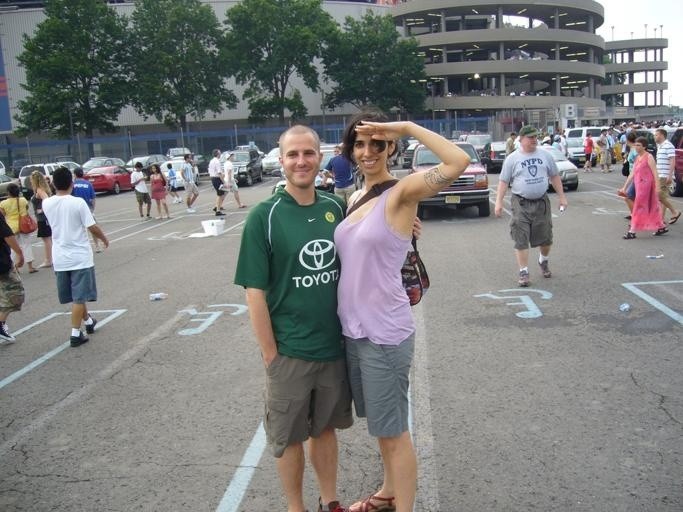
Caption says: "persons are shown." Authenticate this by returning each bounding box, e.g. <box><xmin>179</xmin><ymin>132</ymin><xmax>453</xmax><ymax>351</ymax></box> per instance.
<box><xmin>506</xmin><ymin>53</ymin><xmax>549</xmax><ymax>62</ymax></box>
<box><xmin>493</xmin><ymin>125</ymin><xmax>570</xmax><ymax>287</ymax></box>
<box><xmin>494</xmin><ymin>119</ymin><xmax>682</xmax><ymax>241</ymax></box>
<box><xmin>445</xmin><ymin>86</ymin><xmax>548</xmax><ymax>97</ymax></box>
<box><xmin>132</xmin><ymin>142</ymin><xmax>361</xmax><ymax>220</ymax></box>
<box><xmin>331</xmin><ymin>115</ymin><xmax>473</xmax><ymax>511</ymax></box>
<box><xmin>41</xmin><ymin>168</ymin><xmax>110</xmax><ymax>346</ymax></box>
<box><xmin>0</xmin><ymin>167</ymin><xmax>101</xmax><ymax>341</ymax></box>
<box><xmin>229</xmin><ymin>123</ymin><xmax>351</xmax><ymax>511</ymax></box>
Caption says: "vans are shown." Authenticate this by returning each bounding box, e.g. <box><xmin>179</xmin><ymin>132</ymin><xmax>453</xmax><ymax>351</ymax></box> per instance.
<box><xmin>564</xmin><ymin>126</ymin><xmax>628</xmax><ymax>165</ymax></box>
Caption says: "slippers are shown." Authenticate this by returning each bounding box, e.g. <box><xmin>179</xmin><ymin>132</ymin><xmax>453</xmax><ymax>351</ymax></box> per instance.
<box><xmin>29</xmin><ymin>263</ymin><xmax>53</xmax><ymax>273</ymax></box>
<box><xmin>668</xmin><ymin>212</ymin><xmax>681</xmax><ymax>224</ymax></box>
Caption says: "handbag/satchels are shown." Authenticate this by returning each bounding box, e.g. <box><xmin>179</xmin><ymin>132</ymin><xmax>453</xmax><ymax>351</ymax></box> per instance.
<box><xmin>401</xmin><ymin>251</ymin><xmax>429</xmax><ymax>306</ymax></box>
<box><xmin>622</xmin><ymin>160</ymin><xmax>629</xmax><ymax>177</ymax></box>
<box><xmin>19</xmin><ymin>214</ymin><xmax>37</xmax><ymax>234</ymax></box>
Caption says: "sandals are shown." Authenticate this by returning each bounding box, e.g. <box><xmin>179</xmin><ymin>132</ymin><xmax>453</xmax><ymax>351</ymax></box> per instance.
<box><xmin>349</xmin><ymin>493</ymin><xmax>395</xmax><ymax>512</ymax></box>
<box><xmin>653</xmin><ymin>226</ymin><xmax>669</xmax><ymax>235</ymax></box>
<box><xmin>623</xmin><ymin>231</ymin><xmax>636</xmax><ymax>239</ymax></box>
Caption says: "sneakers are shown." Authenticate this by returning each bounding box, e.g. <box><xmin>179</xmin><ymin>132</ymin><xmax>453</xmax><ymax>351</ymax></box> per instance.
<box><xmin>96</xmin><ymin>246</ymin><xmax>104</xmax><ymax>253</ymax></box>
<box><xmin>0</xmin><ymin>321</ymin><xmax>16</xmax><ymax>342</ymax></box>
<box><xmin>625</xmin><ymin>216</ymin><xmax>632</xmax><ymax>219</ymax></box>
<box><xmin>538</xmin><ymin>258</ymin><xmax>551</xmax><ymax>278</ymax></box>
<box><xmin>213</xmin><ymin>207</ymin><xmax>226</xmax><ymax>216</ymax></box>
<box><xmin>186</xmin><ymin>205</ymin><xmax>196</xmax><ymax>213</ymax></box>
<box><xmin>140</xmin><ymin>215</ymin><xmax>170</xmax><ymax>221</ymax></box>
<box><xmin>85</xmin><ymin>318</ymin><xmax>97</xmax><ymax>334</ymax></box>
<box><xmin>70</xmin><ymin>330</ymin><xmax>89</xmax><ymax>347</ymax></box>
<box><xmin>518</xmin><ymin>270</ymin><xmax>529</xmax><ymax>287</ymax></box>
<box><xmin>173</xmin><ymin>199</ymin><xmax>182</xmax><ymax>203</ymax></box>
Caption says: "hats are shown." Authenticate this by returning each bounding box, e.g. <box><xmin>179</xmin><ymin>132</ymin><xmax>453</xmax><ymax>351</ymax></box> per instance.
<box><xmin>541</xmin><ymin>134</ymin><xmax>560</xmax><ymax>142</ymax></box>
<box><xmin>519</xmin><ymin>125</ymin><xmax>539</xmax><ymax>136</ymax></box>
<box><xmin>226</xmin><ymin>152</ymin><xmax>235</xmax><ymax>160</ymax></box>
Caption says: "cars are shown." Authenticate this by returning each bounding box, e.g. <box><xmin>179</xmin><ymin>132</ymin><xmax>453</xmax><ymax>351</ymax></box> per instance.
<box><xmin>269</xmin><ymin>149</ymin><xmax>338</xmax><ymax>194</ymax></box>
<box><xmin>565</xmin><ymin>139</ymin><xmax>597</xmax><ymax>168</ymax></box>
<box><xmin>457</xmin><ymin>133</ymin><xmax>578</xmax><ymax>195</ymax></box>
<box><xmin>403</xmin><ymin>138</ymin><xmax>424</xmax><ymax>168</ymax></box>
<box><xmin>221</xmin><ymin>145</ymin><xmax>280</xmax><ymax>187</ymax></box>
<box><xmin>0</xmin><ymin>175</ymin><xmax>20</xmax><ymax>199</ymax></box>
<box><xmin>52</xmin><ymin>161</ymin><xmax>80</xmax><ymax>174</ymax></box>
<box><xmin>81</xmin><ymin>146</ymin><xmax>200</xmax><ymax>194</ymax></box>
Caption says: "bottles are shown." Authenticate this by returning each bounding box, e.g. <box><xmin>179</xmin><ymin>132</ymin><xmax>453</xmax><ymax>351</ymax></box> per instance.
<box><xmin>149</xmin><ymin>293</ymin><xmax>168</xmax><ymax>300</ymax></box>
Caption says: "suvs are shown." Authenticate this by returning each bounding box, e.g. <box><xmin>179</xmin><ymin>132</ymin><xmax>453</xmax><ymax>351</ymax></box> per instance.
<box><xmin>409</xmin><ymin>141</ymin><xmax>492</xmax><ymax>222</ymax></box>
<box><xmin>627</xmin><ymin>129</ymin><xmax>675</xmax><ymax>162</ymax></box>
<box><xmin>668</xmin><ymin>128</ymin><xmax>682</xmax><ymax>196</ymax></box>
<box><xmin>17</xmin><ymin>162</ymin><xmax>74</xmax><ymax>195</ymax></box>
<box><xmin>0</xmin><ymin>161</ymin><xmax>6</xmax><ymax>175</ymax></box>
